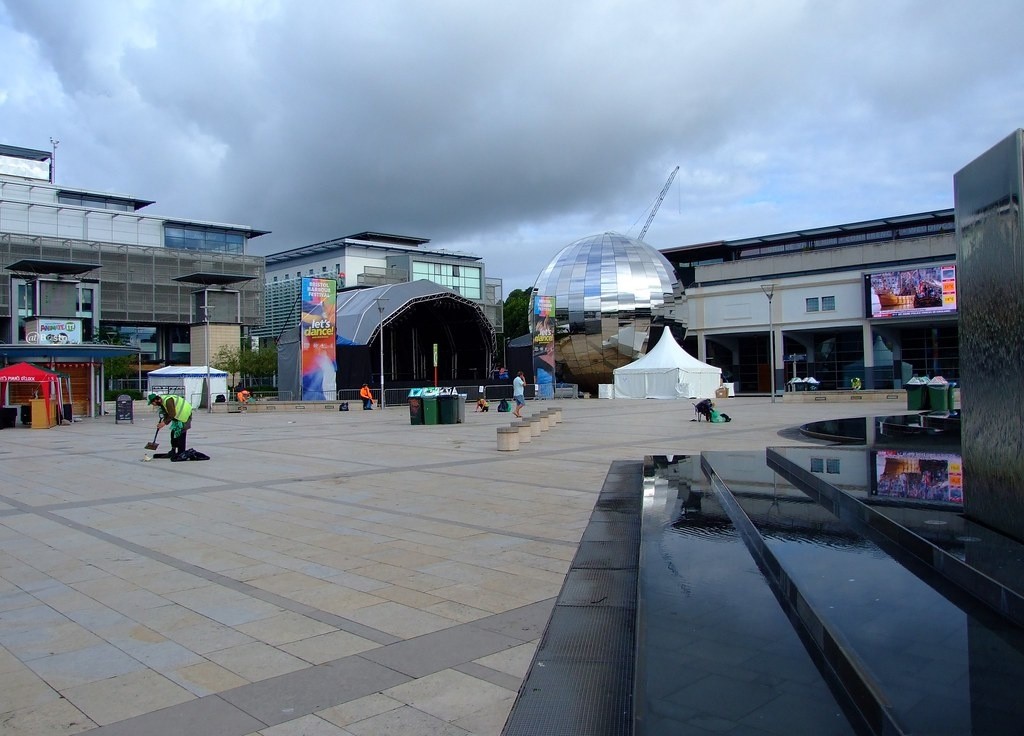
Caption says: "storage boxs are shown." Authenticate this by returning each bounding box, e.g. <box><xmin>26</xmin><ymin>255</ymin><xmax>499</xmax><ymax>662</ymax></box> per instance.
<box><xmin>716</xmin><ymin>388</ymin><xmax>728</xmax><ymax>399</ymax></box>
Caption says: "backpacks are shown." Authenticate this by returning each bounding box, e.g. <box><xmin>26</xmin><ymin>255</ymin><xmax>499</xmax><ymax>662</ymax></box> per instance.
<box><xmin>497</xmin><ymin>398</ymin><xmax>508</xmax><ymax>412</ymax></box>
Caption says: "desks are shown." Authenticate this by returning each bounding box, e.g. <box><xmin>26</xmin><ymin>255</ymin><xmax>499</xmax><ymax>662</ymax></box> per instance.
<box><xmin>28</xmin><ymin>399</ymin><xmax>57</xmax><ymax>429</ymax></box>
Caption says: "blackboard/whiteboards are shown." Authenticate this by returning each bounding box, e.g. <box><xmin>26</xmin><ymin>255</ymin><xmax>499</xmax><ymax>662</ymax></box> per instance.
<box><xmin>116</xmin><ymin>401</ymin><xmax>133</xmax><ymax>420</ymax></box>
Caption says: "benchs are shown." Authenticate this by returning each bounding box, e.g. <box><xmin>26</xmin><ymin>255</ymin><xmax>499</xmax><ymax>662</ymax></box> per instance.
<box><xmin>554</xmin><ymin>387</ymin><xmax>574</xmax><ymax>400</ymax></box>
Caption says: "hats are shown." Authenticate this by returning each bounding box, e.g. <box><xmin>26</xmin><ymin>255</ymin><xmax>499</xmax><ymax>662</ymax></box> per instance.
<box><xmin>362</xmin><ymin>383</ymin><xmax>368</xmax><ymax>387</ymax></box>
<box><xmin>147</xmin><ymin>393</ymin><xmax>156</xmax><ymax>405</ymax></box>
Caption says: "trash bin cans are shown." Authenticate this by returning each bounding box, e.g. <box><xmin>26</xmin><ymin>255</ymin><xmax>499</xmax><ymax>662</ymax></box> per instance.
<box><xmin>903</xmin><ymin>384</ymin><xmax>929</xmax><ymax>411</ymax></box>
<box><xmin>438</xmin><ymin>386</ymin><xmax>462</xmax><ymax>424</ymax></box>
<box><xmin>948</xmin><ymin>383</ymin><xmax>957</xmax><ymax>410</ymax></box>
<box><xmin>407</xmin><ymin>388</ymin><xmax>425</xmax><ymax>425</ymax></box>
<box><xmin>458</xmin><ymin>393</ymin><xmax>467</xmax><ymax>423</ymax></box>
<box><xmin>928</xmin><ymin>383</ymin><xmax>949</xmax><ymax>411</ymax></box>
<box><xmin>421</xmin><ymin>387</ymin><xmax>443</xmax><ymax>425</ymax></box>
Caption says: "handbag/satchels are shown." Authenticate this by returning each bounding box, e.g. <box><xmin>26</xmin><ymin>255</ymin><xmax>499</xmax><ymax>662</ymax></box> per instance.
<box><xmin>339</xmin><ymin>402</ymin><xmax>349</xmax><ymax>411</ymax></box>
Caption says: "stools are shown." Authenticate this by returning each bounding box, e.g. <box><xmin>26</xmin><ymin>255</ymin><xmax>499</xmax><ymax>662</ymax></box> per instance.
<box><xmin>497</xmin><ymin>406</ymin><xmax>563</xmax><ymax>452</ymax></box>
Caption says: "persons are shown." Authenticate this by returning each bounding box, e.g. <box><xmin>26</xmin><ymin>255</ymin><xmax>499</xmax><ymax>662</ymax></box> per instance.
<box><xmin>850</xmin><ymin>377</ymin><xmax>861</xmax><ymax>390</ymax></box>
<box><xmin>146</xmin><ymin>393</ymin><xmax>192</xmax><ymax>457</ymax></box>
<box><xmin>512</xmin><ymin>371</ymin><xmax>526</xmax><ymax>417</ymax></box>
<box><xmin>360</xmin><ymin>383</ymin><xmax>374</xmax><ymax>410</ymax></box>
<box><xmin>695</xmin><ymin>398</ymin><xmax>714</xmax><ymax>421</ymax></box>
<box><xmin>237</xmin><ymin>391</ymin><xmax>255</xmax><ymax>415</ymax></box>
<box><xmin>475</xmin><ymin>396</ymin><xmax>489</xmax><ymax>412</ymax></box>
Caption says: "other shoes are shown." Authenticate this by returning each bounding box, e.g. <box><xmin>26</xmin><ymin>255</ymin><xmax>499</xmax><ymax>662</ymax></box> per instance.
<box><xmin>171</xmin><ymin>450</ymin><xmax>184</xmax><ymax>460</ymax></box>
<box><xmin>363</xmin><ymin>407</ymin><xmax>366</xmax><ymax>410</ymax></box>
<box><xmin>161</xmin><ymin>450</ymin><xmax>175</xmax><ymax>458</ymax></box>
<box><xmin>512</xmin><ymin>411</ymin><xmax>523</xmax><ymax>418</ymax></box>
<box><xmin>367</xmin><ymin>408</ymin><xmax>373</xmax><ymax>410</ymax></box>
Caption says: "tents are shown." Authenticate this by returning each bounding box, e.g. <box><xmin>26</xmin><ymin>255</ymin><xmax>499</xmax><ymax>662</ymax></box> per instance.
<box><xmin>613</xmin><ymin>326</ymin><xmax>722</xmax><ymax>398</ymax></box>
<box><xmin>146</xmin><ymin>365</ymin><xmax>229</xmax><ymax>408</ymax></box>
<box><xmin>0</xmin><ymin>361</ymin><xmax>74</xmax><ymax>429</ymax></box>
